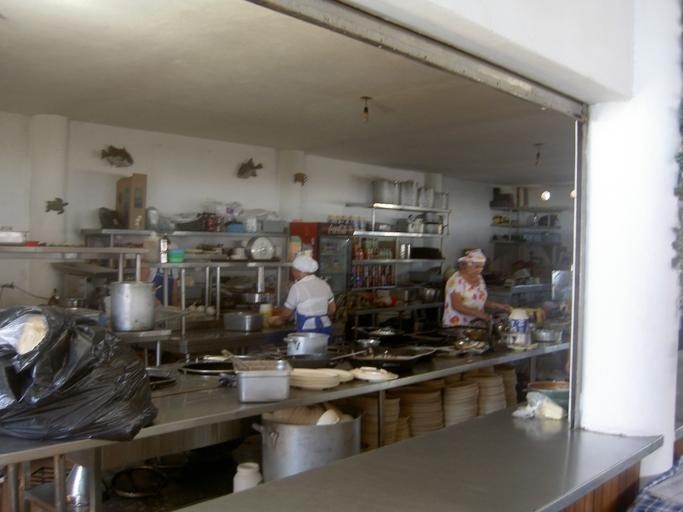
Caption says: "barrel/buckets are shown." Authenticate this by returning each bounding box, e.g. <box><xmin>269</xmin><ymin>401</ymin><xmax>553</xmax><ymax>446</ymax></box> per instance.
<box><xmin>251</xmin><ymin>410</ymin><xmax>362</xmax><ymax>483</ymax></box>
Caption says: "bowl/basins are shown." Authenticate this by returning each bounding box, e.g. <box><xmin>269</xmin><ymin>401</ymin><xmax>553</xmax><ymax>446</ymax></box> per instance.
<box><xmin>260</xmin><ymin>402</ymin><xmax>354</xmax><ymax>426</ymax></box>
<box><xmin>356</xmin><ymin>337</ymin><xmax>381</xmax><ymax>349</ymax></box>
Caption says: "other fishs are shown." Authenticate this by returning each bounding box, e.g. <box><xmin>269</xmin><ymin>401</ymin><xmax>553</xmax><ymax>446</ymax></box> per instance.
<box><xmin>100</xmin><ymin>145</ymin><xmax>134</xmax><ymax>168</ymax></box>
<box><xmin>237</xmin><ymin>158</ymin><xmax>262</xmax><ymax>179</ymax></box>
<box><xmin>45</xmin><ymin>196</ymin><xmax>69</xmax><ymax>215</ymax></box>
<box><xmin>293</xmin><ymin>172</ymin><xmax>307</xmax><ymax>186</ymax></box>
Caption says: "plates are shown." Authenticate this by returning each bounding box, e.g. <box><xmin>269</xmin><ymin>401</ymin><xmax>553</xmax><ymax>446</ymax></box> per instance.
<box><xmin>355</xmin><ymin>365</ymin><xmax>519</xmax><ymax>456</ymax></box>
<box><xmin>288</xmin><ymin>367</ymin><xmax>355</xmax><ymax>391</ymax></box>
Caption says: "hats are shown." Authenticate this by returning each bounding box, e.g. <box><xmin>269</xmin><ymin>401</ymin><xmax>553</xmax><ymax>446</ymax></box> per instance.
<box><xmin>292</xmin><ymin>255</ymin><xmax>318</xmax><ymax>273</ymax></box>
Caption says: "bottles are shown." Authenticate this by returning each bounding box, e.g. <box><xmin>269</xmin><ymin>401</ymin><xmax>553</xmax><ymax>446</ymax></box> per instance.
<box><xmin>233</xmin><ymin>461</ymin><xmax>263</xmax><ymax>496</ymax></box>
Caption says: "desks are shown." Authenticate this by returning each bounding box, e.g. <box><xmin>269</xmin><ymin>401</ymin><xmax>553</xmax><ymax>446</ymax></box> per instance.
<box><xmin>348</xmin><ymin>301</ymin><xmax>445</xmax><ymax>341</ymax></box>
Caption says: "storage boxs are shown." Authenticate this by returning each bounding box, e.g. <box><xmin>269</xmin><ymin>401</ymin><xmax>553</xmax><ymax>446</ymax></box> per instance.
<box><xmin>116</xmin><ymin>172</ymin><xmax>148</xmax><ymax>231</ymax></box>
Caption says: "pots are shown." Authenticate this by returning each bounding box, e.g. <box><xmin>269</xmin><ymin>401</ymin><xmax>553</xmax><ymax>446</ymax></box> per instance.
<box><xmin>522</xmin><ymin>379</ymin><xmax>570</xmax><ymax>410</ymax></box>
<box><xmin>282</xmin><ymin>331</ymin><xmax>330</xmax><ymax>360</ymax></box>
<box><xmin>366</xmin><ymin>176</ymin><xmax>450</xmax><ymax>234</ymax></box>
<box><xmin>253</xmin><ymin>416</ymin><xmax>355</xmax><ymax>486</ymax></box>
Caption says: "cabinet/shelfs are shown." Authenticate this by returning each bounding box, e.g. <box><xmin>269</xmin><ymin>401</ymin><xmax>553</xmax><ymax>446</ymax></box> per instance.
<box><xmin>344</xmin><ymin>203</ymin><xmax>453</xmax><ymax>291</ymax></box>
<box><xmin>0</xmin><ymin>228</ymin><xmax>296</xmax><ymax>366</ymax></box>
<box><xmin>489</xmin><ymin>205</ymin><xmax>569</xmax><ymax>246</ymax></box>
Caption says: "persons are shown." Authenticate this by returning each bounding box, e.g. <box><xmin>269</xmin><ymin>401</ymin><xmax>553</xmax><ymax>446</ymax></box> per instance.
<box><xmin>441</xmin><ymin>248</ymin><xmax>510</xmax><ymax>329</ymax></box>
<box><xmin>269</xmin><ymin>254</ymin><xmax>337</xmax><ymax>347</ymax></box>
